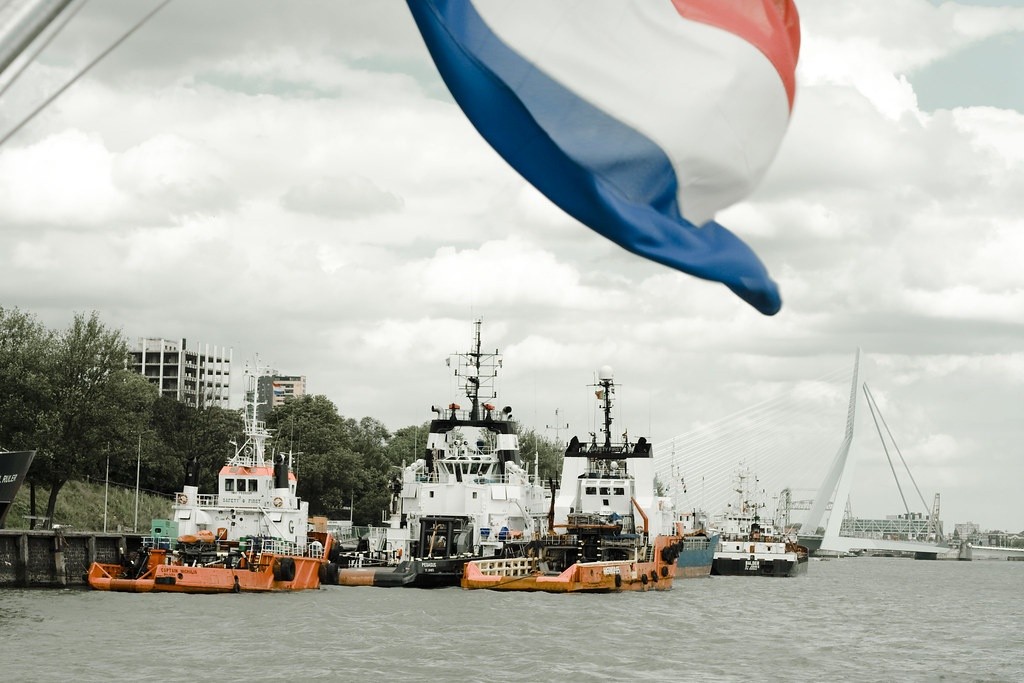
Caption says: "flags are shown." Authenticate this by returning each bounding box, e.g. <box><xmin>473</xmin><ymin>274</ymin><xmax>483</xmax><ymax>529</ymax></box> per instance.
<box><xmin>410</xmin><ymin>0</ymin><xmax>798</xmax><ymax>315</ymax></box>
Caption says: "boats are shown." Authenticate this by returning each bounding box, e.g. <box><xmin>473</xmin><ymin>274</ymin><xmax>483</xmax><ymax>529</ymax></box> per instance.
<box><xmin>670</xmin><ymin>441</ymin><xmax>812</xmax><ymax>578</ymax></box>
<box><xmin>459</xmin><ymin>365</ymin><xmax>686</xmax><ymax>593</ymax></box>
<box><xmin>318</xmin><ymin>316</ymin><xmax>561</xmax><ymax>589</ymax></box>
<box><xmin>87</xmin><ymin>348</ymin><xmax>341</xmax><ymax>591</ymax></box>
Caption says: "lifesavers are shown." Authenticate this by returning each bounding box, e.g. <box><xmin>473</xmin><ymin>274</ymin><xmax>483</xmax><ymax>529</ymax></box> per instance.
<box><xmin>662</xmin><ymin>566</ymin><xmax>668</xmax><ymax>576</ymax></box>
<box><xmin>273</xmin><ymin>496</ymin><xmax>282</xmax><ymax>507</ymax></box>
<box><xmin>281</xmin><ymin>558</ymin><xmax>295</xmax><ymax>580</ymax></box>
<box><xmin>318</xmin><ymin>562</ymin><xmax>339</xmax><ymax>584</ymax></box>
<box><xmin>615</xmin><ymin>574</ymin><xmax>621</xmax><ymax>586</ymax></box>
<box><xmin>651</xmin><ymin>570</ymin><xmax>658</xmax><ymax>582</ymax></box>
<box><xmin>642</xmin><ymin>573</ymin><xmax>648</xmax><ymax>583</ymax></box>
<box><xmin>179</xmin><ymin>493</ymin><xmax>188</xmax><ymax>504</ymax></box>
